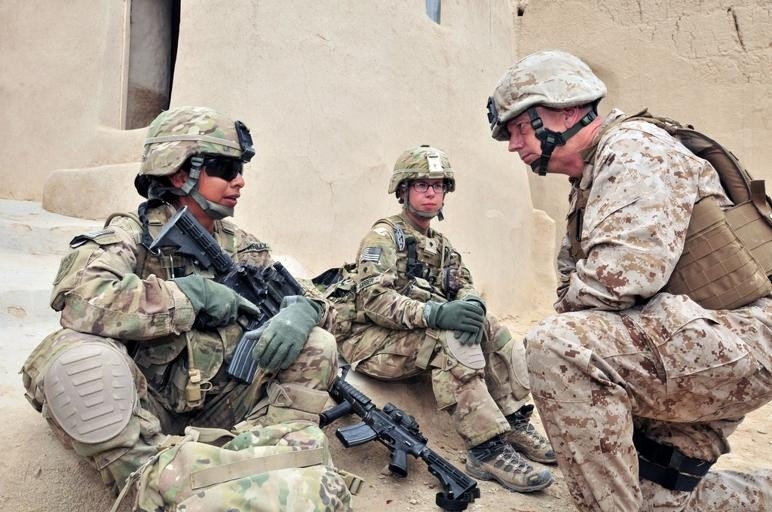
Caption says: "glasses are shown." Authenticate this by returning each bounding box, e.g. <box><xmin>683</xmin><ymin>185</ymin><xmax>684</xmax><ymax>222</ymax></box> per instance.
<box><xmin>202</xmin><ymin>156</ymin><xmax>243</xmax><ymax>182</ymax></box>
<box><xmin>411</xmin><ymin>180</ymin><xmax>444</xmax><ymax>194</ymax></box>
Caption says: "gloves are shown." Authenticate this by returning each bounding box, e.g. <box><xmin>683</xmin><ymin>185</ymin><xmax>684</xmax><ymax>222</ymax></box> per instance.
<box><xmin>170</xmin><ymin>275</ymin><xmax>261</xmax><ymax>329</ymax></box>
<box><xmin>246</xmin><ymin>295</ymin><xmax>323</xmax><ymax>372</ymax></box>
<box><xmin>423</xmin><ymin>300</ymin><xmax>487</xmax><ymax>345</ymax></box>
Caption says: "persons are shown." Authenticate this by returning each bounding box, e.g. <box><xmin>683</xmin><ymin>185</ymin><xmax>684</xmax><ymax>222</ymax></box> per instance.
<box><xmin>485</xmin><ymin>47</ymin><xmax>772</xmax><ymax>512</ymax></box>
<box><xmin>15</xmin><ymin>104</ymin><xmax>359</xmax><ymax>512</ymax></box>
<box><xmin>324</xmin><ymin>143</ymin><xmax>562</xmax><ymax>494</ymax></box>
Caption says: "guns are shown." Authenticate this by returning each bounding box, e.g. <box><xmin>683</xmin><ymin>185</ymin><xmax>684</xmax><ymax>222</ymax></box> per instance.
<box><xmin>319</xmin><ymin>365</ymin><xmax>480</xmax><ymax>512</ymax></box>
<box><xmin>149</xmin><ymin>205</ymin><xmax>376</xmax><ymax>427</ymax></box>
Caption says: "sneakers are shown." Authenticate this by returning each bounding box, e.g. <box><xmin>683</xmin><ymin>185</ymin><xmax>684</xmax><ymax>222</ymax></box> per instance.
<box><xmin>509</xmin><ymin>406</ymin><xmax>558</xmax><ymax>465</ymax></box>
<box><xmin>467</xmin><ymin>436</ymin><xmax>552</xmax><ymax>492</ymax></box>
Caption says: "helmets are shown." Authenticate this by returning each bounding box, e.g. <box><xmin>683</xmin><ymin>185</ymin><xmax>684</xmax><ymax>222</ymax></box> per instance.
<box><xmin>388</xmin><ymin>145</ymin><xmax>456</xmax><ymax>194</ymax></box>
<box><xmin>489</xmin><ymin>52</ymin><xmax>607</xmax><ymax>140</ymax></box>
<box><xmin>134</xmin><ymin>107</ymin><xmax>255</xmax><ymax>197</ymax></box>
